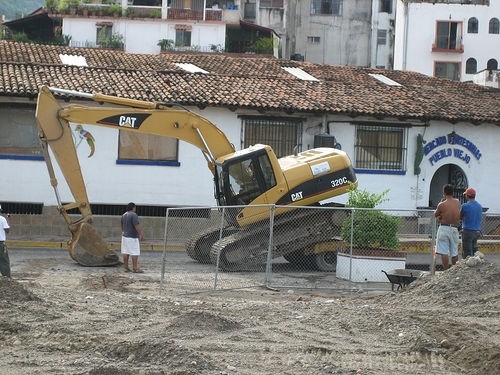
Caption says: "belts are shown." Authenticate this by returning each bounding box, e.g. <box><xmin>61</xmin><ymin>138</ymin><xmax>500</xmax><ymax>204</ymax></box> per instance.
<box><xmin>441</xmin><ymin>224</ymin><xmax>457</xmax><ymax>227</ymax></box>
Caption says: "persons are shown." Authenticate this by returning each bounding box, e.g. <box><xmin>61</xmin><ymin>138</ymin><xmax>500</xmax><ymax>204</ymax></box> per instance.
<box><xmin>460</xmin><ymin>188</ymin><xmax>482</xmax><ymax>259</ymax></box>
<box><xmin>0</xmin><ymin>204</ymin><xmax>11</xmax><ymax>277</ymax></box>
<box><xmin>435</xmin><ymin>184</ymin><xmax>461</xmax><ymax>270</ymax></box>
<box><xmin>121</xmin><ymin>202</ymin><xmax>144</xmax><ymax>273</ymax></box>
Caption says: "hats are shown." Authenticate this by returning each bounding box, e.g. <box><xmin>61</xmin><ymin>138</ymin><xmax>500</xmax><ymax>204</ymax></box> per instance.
<box><xmin>464</xmin><ymin>188</ymin><xmax>476</xmax><ymax>197</ymax></box>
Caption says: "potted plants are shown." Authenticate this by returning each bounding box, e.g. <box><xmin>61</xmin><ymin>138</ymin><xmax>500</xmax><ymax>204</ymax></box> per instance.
<box><xmin>335</xmin><ymin>178</ymin><xmax>407</xmax><ymax>283</ymax></box>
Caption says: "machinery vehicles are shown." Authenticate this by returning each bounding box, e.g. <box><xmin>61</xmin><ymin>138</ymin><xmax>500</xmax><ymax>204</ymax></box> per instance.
<box><xmin>35</xmin><ymin>84</ymin><xmax>359</xmax><ymax>273</ymax></box>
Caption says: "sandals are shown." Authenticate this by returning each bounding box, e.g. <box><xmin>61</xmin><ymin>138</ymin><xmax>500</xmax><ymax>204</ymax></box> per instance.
<box><xmin>132</xmin><ymin>269</ymin><xmax>144</xmax><ymax>273</ymax></box>
<box><xmin>124</xmin><ymin>268</ymin><xmax>131</xmax><ymax>272</ymax></box>
